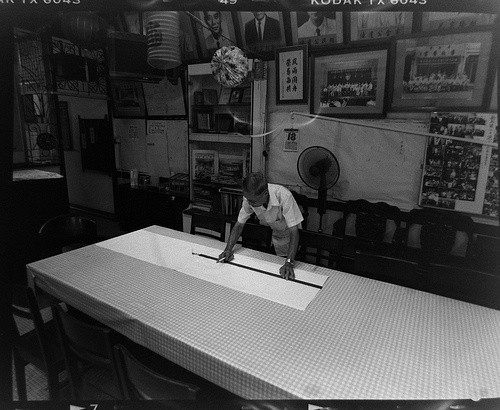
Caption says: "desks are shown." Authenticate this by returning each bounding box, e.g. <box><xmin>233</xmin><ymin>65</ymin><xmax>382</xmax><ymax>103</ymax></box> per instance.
<box><xmin>23</xmin><ymin>221</ymin><xmax>500</xmax><ymax>401</ymax></box>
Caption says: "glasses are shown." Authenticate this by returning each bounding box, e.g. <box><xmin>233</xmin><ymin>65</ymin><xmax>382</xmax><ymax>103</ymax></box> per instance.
<box><xmin>244</xmin><ymin>195</ymin><xmax>256</xmax><ymax>205</ymax></box>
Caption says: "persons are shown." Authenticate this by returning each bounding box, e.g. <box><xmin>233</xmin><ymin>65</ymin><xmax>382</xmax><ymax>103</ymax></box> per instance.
<box><xmin>245</xmin><ymin>11</ymin><xmax>282</xmax><ymax>54</ymax></box>
<box><xmin>322</xmin><ymin>81</ymin><xmax>376</xmax><ymax>107</ymax></box>
<box><xmin>204</xmin><ymin>10</ymin><xmax>232</xmax><ymax>48</ymax></box>
<box><xmin>402</xmin><ymin>71</ymin><xmax>468</xmax><ymax>92</ymax></box>
<box><xmin>298</xmin><ymin>11</ymin><xmax>337</xmax><ymax>39</ymax></box>
<box><xmin>218</xmin><ymin>173</ymin><xmax>306</xmax><ymax>281</ymax></box>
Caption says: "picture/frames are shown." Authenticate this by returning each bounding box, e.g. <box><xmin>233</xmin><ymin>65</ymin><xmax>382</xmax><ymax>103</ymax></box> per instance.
<box><xmin>236</xmin><ymin>11</ymin><xmax>289</xmax><ymax>54</ymax></box>
<box><xmin>145</xmin><ymin>70</ymin><xmax>186</xmax><ymax>121</ymax></box>
<box><xmin>386</xmin><ymin>24</ymin><xmax>498</xmax><ymax>115</ymax></box>
<box><xmin>192</xmin><ymin>12</ymin><xmax>240</xmax><ymax>56</ymax></box>
<box><xmin>346</xmin><ymin>7</ymin><xmax>419</xmax><ymax>42</ymax></box>
<box><xmin>109</xmin><ymin>79</ymin><xmax>144</xmax><ymax>121</ymax></box>
<box><xmin>308</xmin><ymin>43</ymin><xmax>393</xmax><ymax>119</ymax></box>
<box><xmin>416</xmin><ymin>5</ymin><xmax>498</xmax><ymax>28</ymax></box>
<box><xmin>285</xmin><ymin>7</ymin><xmax>346</xmax><ymax>47</ymax></box>
<box><xmin>276</xmin><ymin>41</ymin><xmax>310</xmax><ymax>105</ymax></box>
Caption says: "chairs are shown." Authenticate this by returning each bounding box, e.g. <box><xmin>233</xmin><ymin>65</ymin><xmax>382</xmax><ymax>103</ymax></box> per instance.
<box><xmin>286</xmin><ymin>193</ymin><xmax>500</xmax><ymax>311</ymax></box>
<box><xmin>9</xmin><ymin>284</ymin><xmax>65</xmax><ymax>401</ymax></box>
<box><xmin>29</xmin><ymin>213</ymin><xmax>100</xmax><ymax>258</ymax></box>
<box><xmin>52</xmin><ymin>297</ymin><xmax>130</xmax><ymax>402</ymax></box>
<box><xmin>113</xmin><ymin>342</ymin><xmax>203</xmax><ymax>401</ymax></box>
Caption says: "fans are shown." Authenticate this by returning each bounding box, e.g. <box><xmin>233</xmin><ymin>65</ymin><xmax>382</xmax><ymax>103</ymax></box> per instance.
<box><xmin>296</xmin><ymin>145</ymin><xmax>341</xmax><ymax>264</ymax></box>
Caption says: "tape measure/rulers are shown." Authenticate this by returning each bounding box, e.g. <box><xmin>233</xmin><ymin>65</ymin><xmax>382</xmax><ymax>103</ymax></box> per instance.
<box><xmin>199</xmin><ymin>254</ymin><xmax>323</xmax><ymax>289</ymax></box>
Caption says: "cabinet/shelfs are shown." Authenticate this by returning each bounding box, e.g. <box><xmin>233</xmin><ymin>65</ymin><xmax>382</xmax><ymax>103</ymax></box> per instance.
<box><xmin>187</xmin><ymin>59</ymin><xmax>268</xmax><ymax>211</ymax></box>
<box><xmin>109</xmin><ymin>172</ymin><xmax>188</xmax><ymax>230</ymax></box>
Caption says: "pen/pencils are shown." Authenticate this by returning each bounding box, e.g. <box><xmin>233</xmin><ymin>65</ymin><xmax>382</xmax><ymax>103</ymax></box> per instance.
<box><xmin>219</xmin><ymin>253</ymin><xmax>233</xmax><ymax>262</ymax></box>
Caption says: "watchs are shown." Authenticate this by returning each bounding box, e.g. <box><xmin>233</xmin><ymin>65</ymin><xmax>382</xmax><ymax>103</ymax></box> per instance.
<box><xmin>285</xmin><ymin>259</ymin><xmax>295</xmax><ymax>265</ymax></box>
